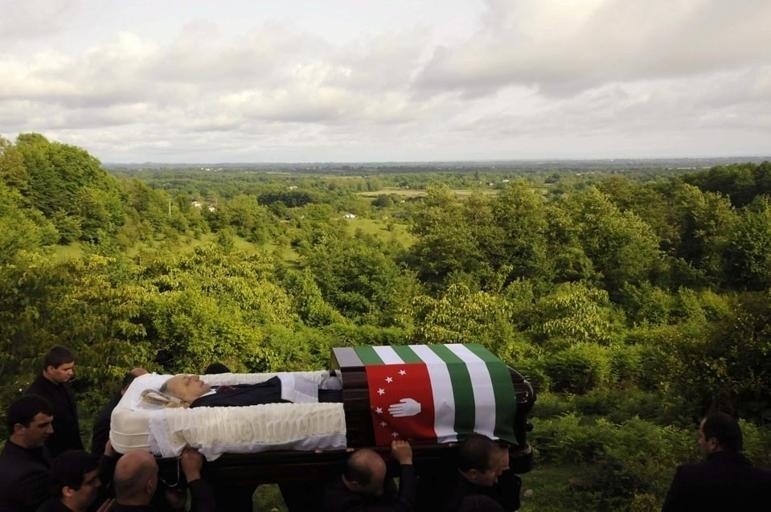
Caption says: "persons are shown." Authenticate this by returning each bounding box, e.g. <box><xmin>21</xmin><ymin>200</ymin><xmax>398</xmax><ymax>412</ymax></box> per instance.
<box><xmin>663</xmin><ymin>409</ymin><xmax>771</xmax><ymax>512</ymax></box>
<box><xmin>27</xmin><ymin>443</ymin><xmax>120</xmax><ymax>512</ymax></box>
<box><xmin>388</xmin><ymin>398</ymin><xmax>424</xmax><ymax>418</ymax></box>
<box><xmin>318</xmin><ymin>438</ymin><xmax>420</xmax><ymax>510</ymax></box>
<box><xmin>159</xmin><ymin>372</ymin><xmax>342</xmax><ymax>407</ymax></box>
<box><xmin>89</xmin><ymin>368</ymin><xmax>152</xmax><ymax>459</ymax></box>
<box><xmin>430</xmin><ymin>433</ymin><xmax>523</xmax><ymax>512</ymax></box>
<box><xmin>98</xmin><ymin>448</ymin><xmax>217</xmax><ymax>512</ymax></box>
<box><xmin>1</xmin><ymin>393</ymin><xmax>69</xmax><ymax>510</ymax></box>
<box><xmin>24</xmin><ymin>346</ymin><xmax>87</xmax><ymax>450</ymax></box>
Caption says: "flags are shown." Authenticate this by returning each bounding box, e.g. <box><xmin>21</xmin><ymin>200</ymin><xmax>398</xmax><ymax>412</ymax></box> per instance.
<box><xmin>354</xmin><ymin>342</ymin><xmax>520</xmax><ymax>447</ymax></box>
